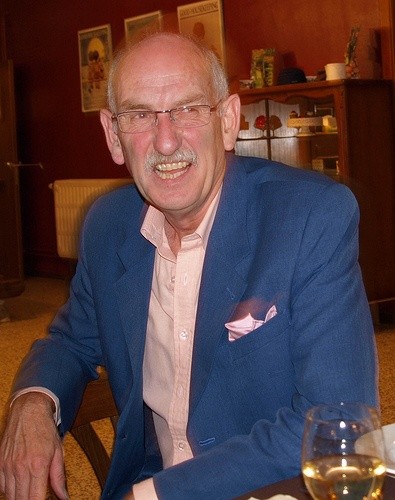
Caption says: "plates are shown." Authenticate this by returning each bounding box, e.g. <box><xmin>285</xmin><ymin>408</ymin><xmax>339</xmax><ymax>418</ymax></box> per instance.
<box><xmin>354</xmin><ymin>422</ymin><xmax>395</xmax><ymax>480</ymax></box>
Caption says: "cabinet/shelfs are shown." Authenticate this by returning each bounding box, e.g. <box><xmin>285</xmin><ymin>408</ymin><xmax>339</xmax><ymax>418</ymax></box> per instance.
<box><xmin>226</xmin><ymin>78</ymin><xmax>395</xmax><ymax>325</ymax></box>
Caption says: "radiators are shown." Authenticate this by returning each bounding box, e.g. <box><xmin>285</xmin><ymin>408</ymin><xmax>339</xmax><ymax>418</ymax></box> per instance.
<box><xmin>53</xmin><ymin>178</ymin><xmax>135</xmax><ymax>259</ymax></box>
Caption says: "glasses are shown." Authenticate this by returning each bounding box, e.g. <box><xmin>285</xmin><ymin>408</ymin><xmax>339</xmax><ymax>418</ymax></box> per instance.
<box><xmin>111</xmin><ymin>99</ymin><xmax>222</xmax><ymax>135</ymax></box>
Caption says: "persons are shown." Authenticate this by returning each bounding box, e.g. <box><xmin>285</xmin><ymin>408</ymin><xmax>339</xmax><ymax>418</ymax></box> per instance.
<box><xmin>0</xmin><ymin>33</ymin><xmax>380</xmax><ymax>500</ymax></box>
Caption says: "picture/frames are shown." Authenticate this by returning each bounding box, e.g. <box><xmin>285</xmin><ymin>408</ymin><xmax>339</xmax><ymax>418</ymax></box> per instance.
<box><xmin>78</xmin><ymin>25</ymin><xmax>113</xmax><ymax>112</ymax></box>
<box><xmin>124</xmin><ymin>10</ymin><xmax>161</xmax><ymax>43</ymax></box>
<box><xmin>177</xmin><ymin>0</ymin><xmax>226</xmax><ymax>80</ymax></box>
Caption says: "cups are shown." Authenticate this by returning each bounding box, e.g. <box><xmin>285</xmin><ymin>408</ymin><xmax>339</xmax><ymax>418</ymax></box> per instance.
<box><xmin>324</xmin><ymin>63</ymin><xmax>347</xmax><ymax>80</ymax></box>
<box><xmin>300</xmin><ymin>401</ymin><xmax>386</xmax><ymax>500</ymax></box>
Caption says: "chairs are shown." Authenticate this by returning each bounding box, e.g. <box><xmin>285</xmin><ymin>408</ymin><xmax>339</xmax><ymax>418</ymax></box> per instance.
<box><xmin>49</xmin><ymin>374</ymin><xmax>121</xmax><ymax>500</ymax></box>
